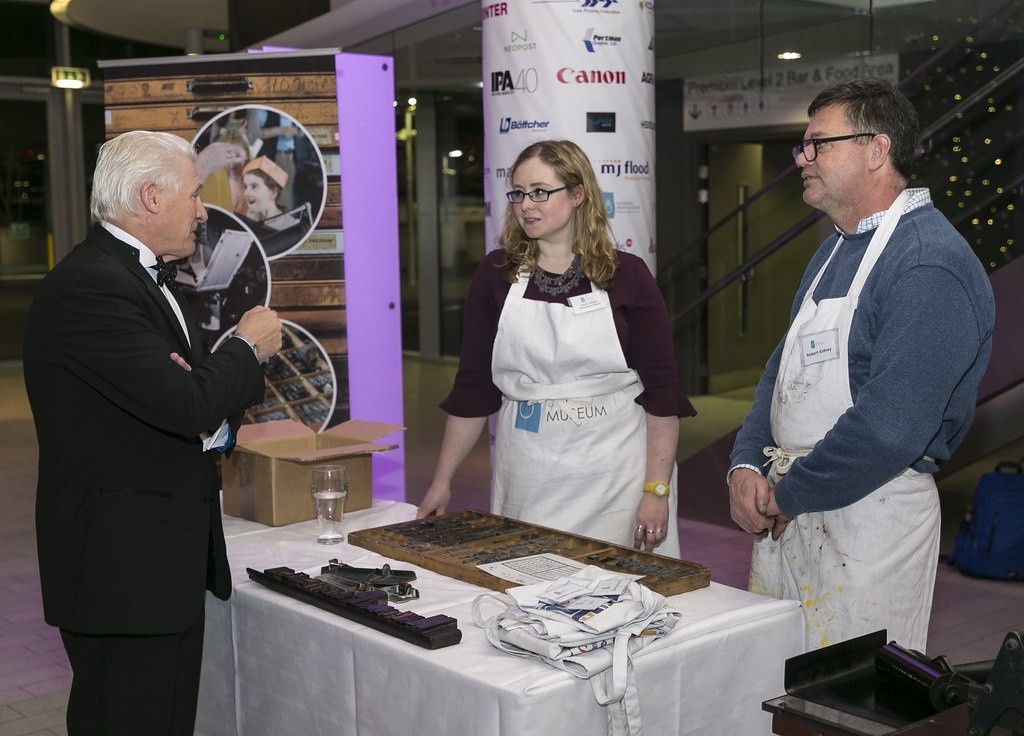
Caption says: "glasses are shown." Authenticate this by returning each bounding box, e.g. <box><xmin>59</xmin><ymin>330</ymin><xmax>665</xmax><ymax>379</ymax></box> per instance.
<box><xmin>506</xmin><ymin>184</ymin><xmax>579</xmax><ymax>203</ymax></box>
<box><xmin>792</xmin><ymin>132</ymin><xmax>877</xmax><ymax>162</ymax></box>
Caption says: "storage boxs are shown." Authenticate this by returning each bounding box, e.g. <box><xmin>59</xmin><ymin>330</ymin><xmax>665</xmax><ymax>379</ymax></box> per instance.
<box><xmin>219</xmin><ymin>418</ymin><xmax>408</xmax><ymax>528</ymax></box>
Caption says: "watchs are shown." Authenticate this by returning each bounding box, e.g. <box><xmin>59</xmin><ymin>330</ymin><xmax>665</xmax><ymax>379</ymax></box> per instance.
<box><xmin>643</xmin><ymin>481</ymin><xmax>670</xmax><ymax>497</ymax></box>
<box><xmin>236</xmin><ymin>333</ymin><xmax>263</xmax><ymax>362</ymax></box>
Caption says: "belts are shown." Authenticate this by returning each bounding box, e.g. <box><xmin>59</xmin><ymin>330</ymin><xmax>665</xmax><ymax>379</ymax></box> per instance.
<box><xmin>277</xmin><ymin>149</ymin><xmax>294</xmax><ymax>153</ymax></box>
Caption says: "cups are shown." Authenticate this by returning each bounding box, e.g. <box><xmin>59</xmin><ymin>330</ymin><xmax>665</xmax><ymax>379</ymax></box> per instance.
<box><xmin>311</xmin><ymin>465</ymin><xmax>348</xmax><ymax>544</ymax></box>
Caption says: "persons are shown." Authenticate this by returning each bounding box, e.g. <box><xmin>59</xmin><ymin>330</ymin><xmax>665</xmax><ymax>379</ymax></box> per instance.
<box><xmin>723</xmin><ymin>75</ymin><xmax>999</xmax><ymax>656</ymax></box>
<box><xmin>418</xmin><ymin>139</ymin><xmax>699</xmax><ymax>560</ymax></box>
<box><xmin>20</xmin><ymin>130</ymin><xmax>285</xmax><ymax>736</ymax></box>
<box><xmin>195</xmin><ymin>108</ymin><xmax>323</xmax><ymax>231</ymax></box>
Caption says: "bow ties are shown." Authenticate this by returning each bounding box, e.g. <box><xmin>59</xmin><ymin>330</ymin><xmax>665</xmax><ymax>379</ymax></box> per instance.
<box><xmin>150</xmin><ymin>255</ymin><xmax>177</xmax><ymax>287</ymax></box>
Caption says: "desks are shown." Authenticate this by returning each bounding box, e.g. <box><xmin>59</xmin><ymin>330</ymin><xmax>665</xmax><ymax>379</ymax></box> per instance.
<box><xmin>193</xmin><ymin>491</ymin><xmax>806</xmax><ymax>736</ymax></box>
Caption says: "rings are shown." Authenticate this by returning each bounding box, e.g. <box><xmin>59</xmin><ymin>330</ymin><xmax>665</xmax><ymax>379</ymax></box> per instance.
<box><xmin>645</xmin><ymin>529</ymin><xmax>655</xmax><ymax>533</ymax></box>
<box><xmin>226</xmin><ymin>151</ymin><xmax>231</xmax><ymax>157</ymax></box>
<box><xmin>655</xmin><ymin>529</ymin><xmax>663</xmax><ymax>532</ymax></box>
<box><xmin>636</xmin><ymin>525</ymin><xmax>644</xmax><ymax>532</ymax></box>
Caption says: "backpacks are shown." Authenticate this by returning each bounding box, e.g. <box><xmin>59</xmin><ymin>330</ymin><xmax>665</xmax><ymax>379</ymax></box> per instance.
<box><xmin>954</xmin><ymin>461</ymin><xmax>1024</xmax><ymax>581</ymax></box>
